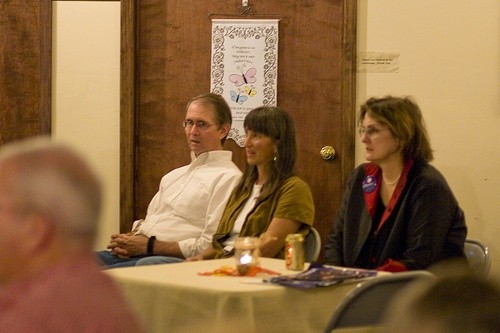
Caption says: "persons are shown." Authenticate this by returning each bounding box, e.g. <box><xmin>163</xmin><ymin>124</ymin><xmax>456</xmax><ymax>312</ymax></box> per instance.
<box><xmin>95</xmin><ymin>93</ymin><xmax>244</xmax><ymax>269</ymax></box>
<box><xmin>322</xmin><ymin>96</ymin><xmax>468</xmax><ymax>273</ymax></box>
<box><xmin>135</xmin><ymin>107</ymin><xmax>315</xmax><ymax>266</ymax></box>
<box><xmin>0</xmin><ymin>136</ymin><xmax>147</xmax><ymax>333</ymax></box>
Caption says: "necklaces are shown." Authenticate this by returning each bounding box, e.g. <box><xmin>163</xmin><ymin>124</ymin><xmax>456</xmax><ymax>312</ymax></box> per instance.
<box><xmin>383</xmin><ymin>168</ymin><xmax>403</xmax><ymax>184</ymax></box>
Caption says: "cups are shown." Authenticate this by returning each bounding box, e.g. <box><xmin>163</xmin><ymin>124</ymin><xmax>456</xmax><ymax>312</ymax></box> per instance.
<box><xmin>234</xmin><ymin>236</ymin><xmax>261</xmax><ymax>276</ymax></box>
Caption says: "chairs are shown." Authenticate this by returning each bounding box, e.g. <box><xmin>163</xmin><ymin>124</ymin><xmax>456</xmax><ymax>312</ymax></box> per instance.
<box><xmin>323</xmin><ymin>270</ymin><xmax>435</xmax><ymax>333</ymax></box>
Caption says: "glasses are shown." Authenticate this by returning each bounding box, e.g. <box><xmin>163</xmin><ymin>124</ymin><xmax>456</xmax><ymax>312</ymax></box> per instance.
<box><xmin>183</xmin><ymin>119</ymin><xmax>218</xmax><ymax>130</ymax></box>
<box><xmin>359</xmin><ymin>126</ymin><xmax>392</xmax><ymax>137</ymax></box>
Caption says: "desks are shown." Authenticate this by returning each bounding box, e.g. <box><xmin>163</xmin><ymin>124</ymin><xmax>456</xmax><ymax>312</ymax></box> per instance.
<box><xmin>101</xmin><ymin>258</ymin><xmax>395</xmax><ymax>333</ymax></box>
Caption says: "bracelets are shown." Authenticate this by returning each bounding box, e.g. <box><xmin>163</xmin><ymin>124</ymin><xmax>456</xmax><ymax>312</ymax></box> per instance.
<box><xmin>145</xmin><ymin>236</ymin><xmax>157</xmax><ymax>256</ymax></box>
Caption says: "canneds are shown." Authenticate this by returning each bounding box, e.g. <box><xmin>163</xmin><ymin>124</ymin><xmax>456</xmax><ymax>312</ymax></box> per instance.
<box><xmin>285</xmin><ymin>233</ymin><xmax>305</xmax><ymax>272</ymax></box>
